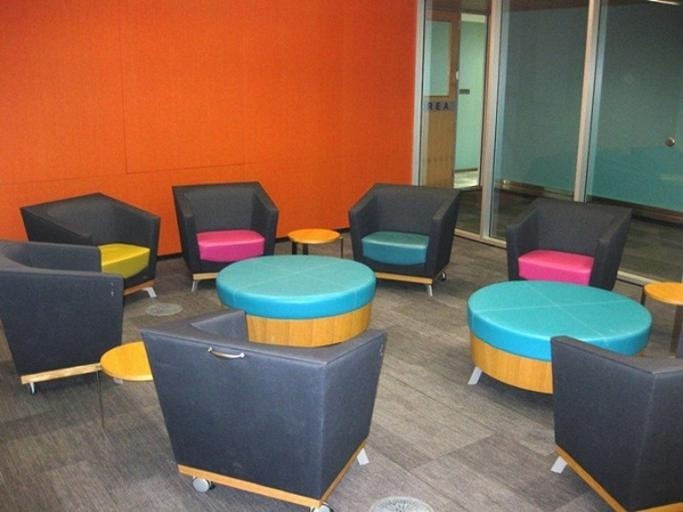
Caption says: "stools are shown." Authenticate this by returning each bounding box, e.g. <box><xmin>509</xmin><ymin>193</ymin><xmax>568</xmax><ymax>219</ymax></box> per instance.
<box><xmin>638</xmin><ymin>281</ymin><xmax>683</xmax><ymax>360</ymax></box>
<box><xmin>286</xmin><ymin>228</ymin><xmax>344</xmax><ymax>260</ymax></box>
<box><xmin>93</xmin><ymin>336</ymin><xmax>154</xmax><ymax>434</ymax></box>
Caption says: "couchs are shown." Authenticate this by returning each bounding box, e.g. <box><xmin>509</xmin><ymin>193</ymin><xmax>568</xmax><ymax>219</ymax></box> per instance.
<box><xmin>547</xmin><ymin>334</ymin><xmax>683</xmax><ymax>512</ymax></box>
<box><xmin>166</xmin><ymin>178</ymin><xmax>281</xmax><ymax>291</ymax></box>
<box><xmin>347</xmin><ymin>178</ymin><xmax>463</xmax><ymax>297</ymax></box>
<box><xmin>136</xmin><ymin>309</ymin><xmax>389</xmax><ymax>512</ymax></box>
<box><xmin>0</xmin><ymin>235</ymin><xmax>124</xmax><ymax>396</ymax></box>
<box><xmin>500</xmin><ymin>195</ymin><xmax>635</xmax><ymax>292</ymax></box>
<box><xmin>16</xmin><ymin>189</ymin><xmax>166</xmax><ymax>300</ymax></box>
